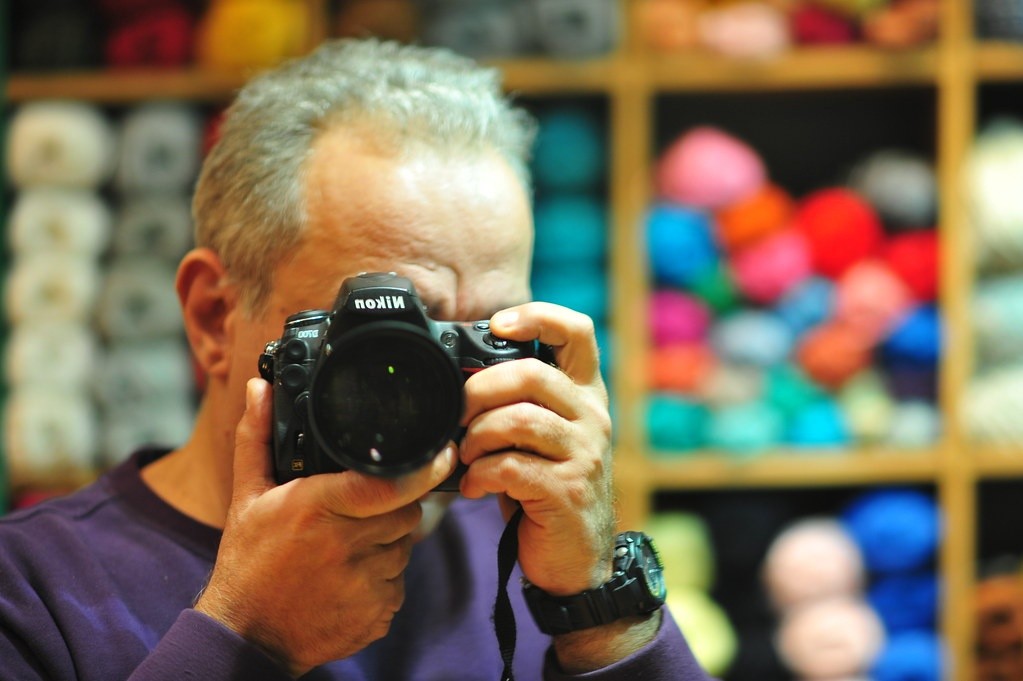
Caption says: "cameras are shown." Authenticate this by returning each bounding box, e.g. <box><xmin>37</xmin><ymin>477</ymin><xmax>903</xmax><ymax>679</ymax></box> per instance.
<box><xmin>270</xmin><ymin>273</ymin><xmax>554</xmax><ymax>493</ymax></box>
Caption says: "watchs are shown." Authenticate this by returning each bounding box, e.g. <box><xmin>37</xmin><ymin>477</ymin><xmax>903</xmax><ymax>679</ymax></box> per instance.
<box><xmin>512</xmin><ymin>530</ymin><xmax>667</xmax><ymax>637</ymax></box>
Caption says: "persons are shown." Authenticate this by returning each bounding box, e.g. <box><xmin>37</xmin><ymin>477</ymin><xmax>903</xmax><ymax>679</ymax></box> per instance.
<box><xmin>1</xmin><ymin>38</ymin><xmax>703</xmax><ymax>680</ymax></box>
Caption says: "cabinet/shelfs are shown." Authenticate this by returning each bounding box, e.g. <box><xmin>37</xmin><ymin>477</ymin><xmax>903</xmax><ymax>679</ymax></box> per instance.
<box><xmin>0</xmin><ymin>0</ymin><xmax>1023</xmax><ymax>681</ymax></box>
